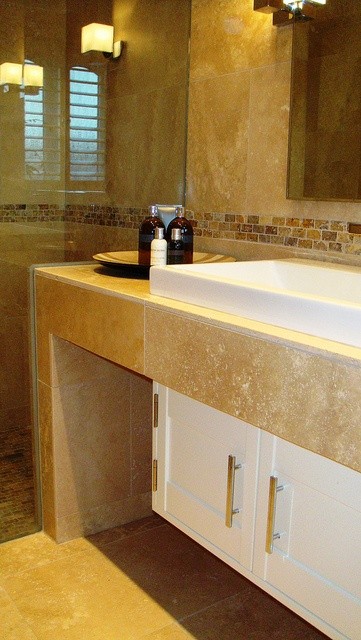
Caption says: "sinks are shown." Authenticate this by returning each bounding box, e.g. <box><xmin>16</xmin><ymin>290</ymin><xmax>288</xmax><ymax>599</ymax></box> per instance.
<box><xmin>150</xmin><ymin>258</ymin><xmax>361</xmax><ymax>352</ymax></box>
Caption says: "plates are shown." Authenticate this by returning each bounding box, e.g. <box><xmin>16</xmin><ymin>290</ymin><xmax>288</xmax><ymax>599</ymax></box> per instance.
<box><xmin>92</xmin><ymin>251</ymin><xmax>237</xmax><ymax>275</ymax></box>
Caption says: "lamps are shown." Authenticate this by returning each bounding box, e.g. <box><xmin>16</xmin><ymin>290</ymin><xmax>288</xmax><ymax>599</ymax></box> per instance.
<box><xmin>294</xmin><ymin>1</ymin><xmax>327</xmax><ymax>22</ymax></box>
<box><xmin>19</xmin><ymin>64</ymin><xmax>44</xmax><ymax>95</ymax></box>
<box><xmin>0</xmin><ymin>62</ymin><xmax>22</xmax><ymax>93</ymax></box>
<box><xmin>253</xmin><ymin>1</ymin><xmax>294</xmax><ymax>26</ymax></box>
<box><xmin>81</xmin><ymin>22</ymin><xmax>124</xmax><ymax>61</ymax></box>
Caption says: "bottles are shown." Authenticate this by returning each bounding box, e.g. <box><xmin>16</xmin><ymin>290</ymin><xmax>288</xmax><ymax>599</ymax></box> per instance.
<box><xmin>168</xmin><ymin>228</ymin><xmax>187</xmax><ymax>265</ymax></box>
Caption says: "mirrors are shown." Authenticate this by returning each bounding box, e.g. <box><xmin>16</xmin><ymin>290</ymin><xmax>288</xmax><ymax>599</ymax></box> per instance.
<box><xmin>286</xmin><ymin>0</ymin><xmax>361</xmax><ymax>203</ymax></box>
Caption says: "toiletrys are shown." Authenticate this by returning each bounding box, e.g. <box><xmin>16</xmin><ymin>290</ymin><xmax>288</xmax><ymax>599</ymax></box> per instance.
<box><xmin>166</xmin><ymin>228</ymin><xmax>185</xmax><ymax>264</ymax></box>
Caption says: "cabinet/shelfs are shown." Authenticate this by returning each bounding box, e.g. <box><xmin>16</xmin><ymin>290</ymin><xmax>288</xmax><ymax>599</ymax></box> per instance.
<box><xmin>151</xmin><ymin>380</ymin><xmax>361</xmax><ymax>640</ymax></box>
